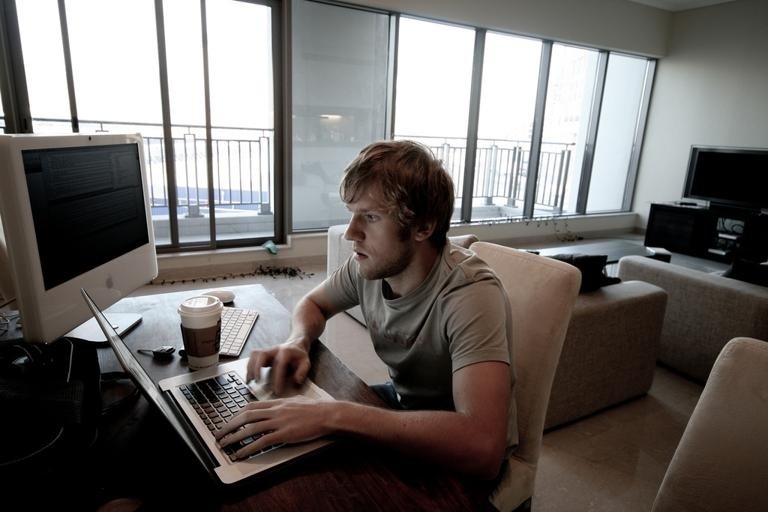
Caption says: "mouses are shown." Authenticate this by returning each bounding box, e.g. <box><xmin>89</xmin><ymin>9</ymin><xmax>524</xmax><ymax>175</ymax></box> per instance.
<box><xmin>206</xmin><ymin>290</ymin><xmax>236</xmax><ymax>304</ymax></box>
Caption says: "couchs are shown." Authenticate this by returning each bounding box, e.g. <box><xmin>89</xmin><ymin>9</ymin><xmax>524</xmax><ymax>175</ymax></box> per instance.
<box><xmin>450</xmin><ymin>233</ymin><xmax>671</xmax><ymax>434</ymax></box>
<box><xmin>615</xmin><ymin>252</ymin><xmax>768</xmax><ymax>383</ymax></box>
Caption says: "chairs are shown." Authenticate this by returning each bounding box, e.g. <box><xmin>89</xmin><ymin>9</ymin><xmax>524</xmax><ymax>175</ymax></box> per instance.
<box><xmin>648</xmin><ymin>335</ymin><xmax>768</xmax><ymax>511</ymax></box>
<box><xmin>463</xmin><ymin>241</ymin><xmax>583</xmax><ymax>511</ymax></box>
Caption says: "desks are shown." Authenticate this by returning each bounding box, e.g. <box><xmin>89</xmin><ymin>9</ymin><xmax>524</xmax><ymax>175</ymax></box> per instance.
<box><xmin>0</xmin><ymin>282</ymin><xmax>504</xmax><ymax>511</ymax></box>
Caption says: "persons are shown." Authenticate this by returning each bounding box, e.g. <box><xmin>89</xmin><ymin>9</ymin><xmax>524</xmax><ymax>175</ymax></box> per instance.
<box><xmin>213</xmin><ymin>138</ymin><xmax>523</xmax><ymax>508</ymax></box>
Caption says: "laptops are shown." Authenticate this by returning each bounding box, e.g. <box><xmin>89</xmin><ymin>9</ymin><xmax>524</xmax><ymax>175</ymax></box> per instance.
<box><xmin>78</xmin><ymin>286</ymin><xmax>349</xmax><ymax>491</ymax></box>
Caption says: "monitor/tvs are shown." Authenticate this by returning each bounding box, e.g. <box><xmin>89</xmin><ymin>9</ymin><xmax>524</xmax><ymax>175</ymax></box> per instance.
<box><xmin>0</xmin><ymin>132</ymin><xmax>159</xmax><ymax>346</ymax></box>
<box><xmin>680</xmin><ymin>144</ymin><xmax>768</xmax><ymax>214</ymax></box>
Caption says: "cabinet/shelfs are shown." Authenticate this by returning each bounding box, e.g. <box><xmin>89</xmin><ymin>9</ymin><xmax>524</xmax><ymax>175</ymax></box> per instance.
<box><xmin>642</xmin><ymin>201</ymin><xmax>768</xmax><ymax>266</ymax></box>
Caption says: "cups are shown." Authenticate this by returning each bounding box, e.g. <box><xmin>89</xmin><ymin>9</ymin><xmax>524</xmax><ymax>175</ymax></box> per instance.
<box><xmin>176</xmin><ymin>294</ymin><xmax>225</xmax><ymax>370</ymax></box>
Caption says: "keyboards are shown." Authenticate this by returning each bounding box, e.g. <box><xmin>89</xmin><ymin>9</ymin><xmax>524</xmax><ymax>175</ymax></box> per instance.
<box><xmin>220</xmin><ymin>306</ymin><xmax>258</xmax><ymax>358</ymax></box>
